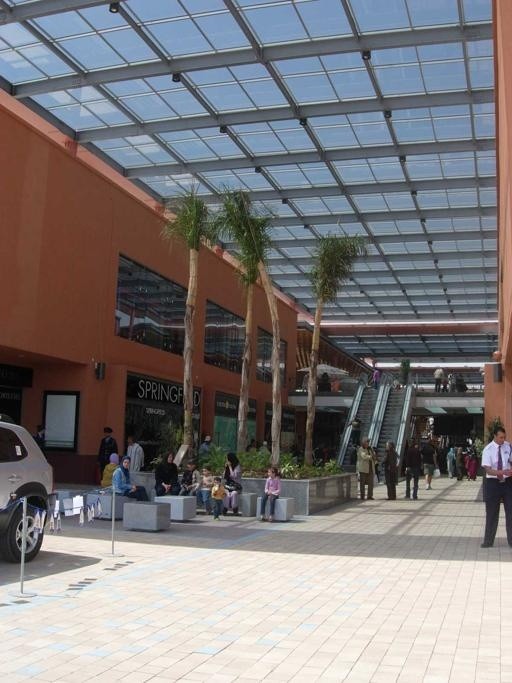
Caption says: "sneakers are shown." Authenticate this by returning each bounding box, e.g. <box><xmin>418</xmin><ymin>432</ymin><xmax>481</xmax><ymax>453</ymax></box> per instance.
<box><xmin>260</xmin><ymin>515</ymin><xmax>273</xmax><ymax>522</ymax></box>
<box><xmin>480</xmin><ymin>542</ymin><xmax>494</xmax><ymax>548</ymax></box>
<box><xmin>212</xmin><ymin>516</ymin><xmax>220</xmax><ymax>522</ymax></box>
<box><xmin>425</xmin><ymin>484</ymin><xmax>430</xmax><ymax>490</ymax></box>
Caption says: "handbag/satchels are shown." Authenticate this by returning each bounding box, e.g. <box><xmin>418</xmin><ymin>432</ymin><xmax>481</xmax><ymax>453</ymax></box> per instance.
<box><xmin>224</xmin><ymin>480</ymin><xmax>242</xmax><ymax>491</ymax></box>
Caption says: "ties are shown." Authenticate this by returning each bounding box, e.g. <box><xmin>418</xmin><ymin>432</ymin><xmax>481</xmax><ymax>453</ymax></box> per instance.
<box><xmin>497</xmin><ymin>445</ymin><xmax>503</xmax><ymax>481</ymax></box>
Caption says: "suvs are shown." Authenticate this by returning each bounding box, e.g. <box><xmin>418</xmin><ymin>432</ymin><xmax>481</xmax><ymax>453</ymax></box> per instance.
<box><xmin>0</xmin><ymin>414</ymin><xmax>54</xmax><ymax>563</ymax></box>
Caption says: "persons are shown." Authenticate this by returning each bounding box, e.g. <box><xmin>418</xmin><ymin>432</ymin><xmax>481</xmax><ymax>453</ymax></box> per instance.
<box><xmin>379</xmin><ymin>439</ymin><xmax>397</xmax><ymax>499</ymax></box>
<box><xmin>287</xmin><ymin>439</ymin><xmax>337</xmax><ymax>467</ymax></box>
<box><xmin>404</xmin><ymin>436</ymin><xmax>419</xmax><ymax>498</ymax></box>
<box><xmin>356</xmin><ymin>437</ymin><xmax>374</xmax><ymax>499</ymax></box>
<box><xmin>258</xmin><ymin>440</ymin><xmax>270</xmax><ymax>454</ymax></box>
<box><xmin>96</xmin><ymin>426</ymin><xmax>118</xmax><ymax>480</ymax></box>
<box><xmin>111</xmin><ymin>455</ymin><xmax>149</xmax><ymax>502</ymax></box>
<box><xmin>259</xmin><ymin>468</ymin><xmax>280</xmax><ymax>522</ymax></box>
<box><xmin>245</xmin><ymin>439</ymin><xmax>255</xmax><ymax>451</ymax></box>
<box><xmin>420</xmin><ymin>441</ymin><xmax>478</xmax><ymax>490</ymax></box>
<box><xmin>100</xmin><ymin>452</ymin><xmax>120</xmax><ymax>486</ymax></box>
<box><xmin>30</xmin><ymin>424</ymin><xmax>46</xmax><ymax>450</ymax></box>
<box><xmin>151</xmin><ymin>450</ymin><xmax>242</xmax><ymax>522</ymax></box>
<box><xmin>371</xmin><ymin>446</ymin><xmax>380</xmax><ymax>483</ymax></box>
<box><xmin>478</xmin><ymin>425</ymin><xmax>511</xmax><ymax>549</ymax></box>
<box><xmin>297</xmin><ymin>361</ymin><xmax>469</xmax><ymax>392</ymax></box>
<box><xmin>198</xmin><ymin>434</ymin><xmax>216</xmax><ymax>457</ymax></box>
<box><xmin>125</xmin><ymin>435</ymin><xmax>145</xmax><ymax>470</ymax></box>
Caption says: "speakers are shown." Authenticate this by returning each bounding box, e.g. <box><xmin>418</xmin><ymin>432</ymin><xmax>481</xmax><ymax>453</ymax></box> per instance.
<box><xmin>96</xmin><ymin>362</ymin><xmax>105</xmax><ymax>380</ymax></box>
<box><xmin>493</xmin><ymin>364</ymin><xmax>502</xmax><ymax>382</ymax></box>
